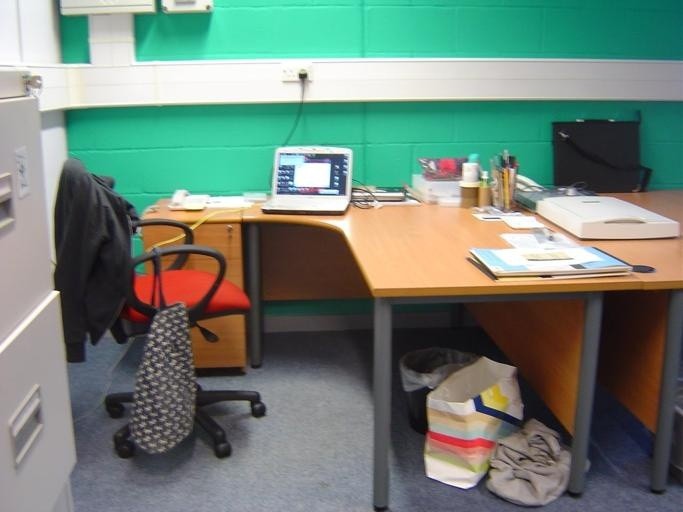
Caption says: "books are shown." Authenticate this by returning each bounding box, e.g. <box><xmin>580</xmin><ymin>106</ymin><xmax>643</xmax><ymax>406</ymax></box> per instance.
<box><xmin>468</xmin><ymin>246</ymin><xmax>634</xmax><ymax>282</ymax></box>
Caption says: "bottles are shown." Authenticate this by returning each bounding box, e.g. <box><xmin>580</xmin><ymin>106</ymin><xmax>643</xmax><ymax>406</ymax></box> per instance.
<box><xmin>478</xmin><ymin>171</ymin><xmax>491</xmax><ymax>209</ymax></box>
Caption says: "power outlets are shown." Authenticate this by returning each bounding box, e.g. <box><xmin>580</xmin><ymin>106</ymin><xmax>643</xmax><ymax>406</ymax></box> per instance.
<box><xmin>280</xmin><ymin>65</ymin><xmax>310</xmax><ymax>84</ymax></box>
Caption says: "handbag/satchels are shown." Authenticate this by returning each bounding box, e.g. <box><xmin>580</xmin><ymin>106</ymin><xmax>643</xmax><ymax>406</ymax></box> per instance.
<box><xmin>423</xmin><ymin>355</ymin><xmax>524</xmax><ymax>489</ymax></box>
<box><xmin>487</xmin><ymin>418</ymin><xmax>590</xmax><ymax>507</ymax></box>
<box><xmin>129</xmin><ymin>303</ymin><xmax>200</xmax><ymax>456</ymax></box>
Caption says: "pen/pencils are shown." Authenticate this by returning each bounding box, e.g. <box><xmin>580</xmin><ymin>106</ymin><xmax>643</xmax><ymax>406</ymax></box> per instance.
<box><xmin>491</xmin><ymin>149</ymin><xmax>520</xmax><ymax>212</ymax></box>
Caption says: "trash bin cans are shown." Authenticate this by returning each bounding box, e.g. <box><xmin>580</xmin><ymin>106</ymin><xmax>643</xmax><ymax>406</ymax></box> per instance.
<box><xmin>397</xmin><ymin>346</ymin><xmax>480</xmax><ymax>435</ymax></box>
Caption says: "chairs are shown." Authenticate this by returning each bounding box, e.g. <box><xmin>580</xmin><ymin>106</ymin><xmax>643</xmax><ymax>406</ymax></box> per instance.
<box><xmin>58</xmin><ymin>157</ymin><xmax>266</xmax><ymax>459</ymax></box>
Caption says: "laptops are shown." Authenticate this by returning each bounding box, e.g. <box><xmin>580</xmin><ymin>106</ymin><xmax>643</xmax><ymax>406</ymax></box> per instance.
<box><xmin>262</xmin><ymin>146</ymin><xmax>353</xmax><ymax>215</ymax></box>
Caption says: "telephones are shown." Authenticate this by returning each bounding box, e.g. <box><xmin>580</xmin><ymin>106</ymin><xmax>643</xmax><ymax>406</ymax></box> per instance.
<box><xmin>168</xmin><ymin>189</ymin><xmax>210</xmax><ymax>211</ymax></box>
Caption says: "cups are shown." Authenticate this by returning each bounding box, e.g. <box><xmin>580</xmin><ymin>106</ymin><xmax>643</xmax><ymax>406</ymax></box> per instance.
<box><xmin>462</xmin><ymin>163</ymin><xmax>479</xmax><ymax>183</ymax></box>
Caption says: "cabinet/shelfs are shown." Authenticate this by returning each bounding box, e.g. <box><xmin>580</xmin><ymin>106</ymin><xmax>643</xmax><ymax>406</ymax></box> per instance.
<box><xmin>0</xmin><ymin>62</ymin><xmax>78</xmax><ymax>512</ymax></box>
